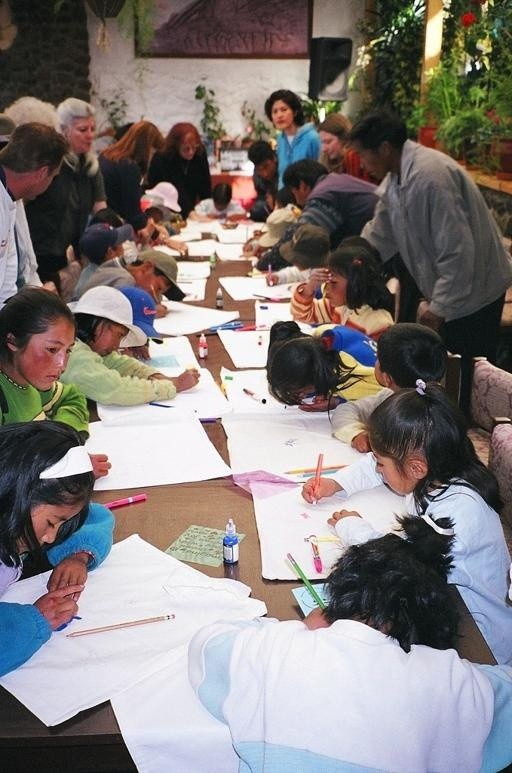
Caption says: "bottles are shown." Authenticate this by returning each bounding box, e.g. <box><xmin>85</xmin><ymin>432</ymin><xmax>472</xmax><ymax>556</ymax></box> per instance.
<box><xmin>223</xmin><ymin>517</ymin><xmax>239</xmax><ymax>564</ymax></box>
<box><xmin>210</xmin><ymin>250</ymin><xmax>216</xmax><ymax>264</ymax></box>
<box><xmin>215</xmin><ymin>287</ymin><xmax>223</xmax><ymax>308</ymax></box>
<box><xmin>198</xmin><ymin>334</ymin><xmax>208</xmax><ymax>358</ymax></box>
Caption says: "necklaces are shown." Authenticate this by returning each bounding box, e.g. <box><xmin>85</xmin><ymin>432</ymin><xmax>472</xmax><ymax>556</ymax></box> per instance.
<box><xmin>179</xmin><ymin>157</ymin><xmax>190</xmax><ymax>176</ymax></box>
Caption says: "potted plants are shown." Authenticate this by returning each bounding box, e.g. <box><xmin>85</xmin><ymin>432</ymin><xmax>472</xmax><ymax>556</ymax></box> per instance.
<box><xmin>405</xmin><ymin>75</ymin><xmax>446</xmax><ymax>149</ymax></box>
<box><xmin>474</xmin><ymin>67</ymin><xmax>512</xmax><ymax>138</ymax></box>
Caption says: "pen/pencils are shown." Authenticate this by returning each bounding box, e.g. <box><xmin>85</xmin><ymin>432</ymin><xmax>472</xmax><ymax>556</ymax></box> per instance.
<box><xmin>253</xmin><ymin>294</ymin><xmax>280</xmax><ymax>302</ymax></box>
<box><xmin>104</xmin><ymin>494</ymin><xmax>146</xmax><ymax>508</ymax></box>
<box><xmin>66</xmin><ymin>614</ymin><xmax>174</xmax><ymax>637</ymax></box>
<box><xmin>150</xmin><ymin>284</ymin><xmax>160</xmax><ymax>305</ymax></box>
<box><xmin>285</xmin><ymin>465</ymin><xmax>349</xmax><ymax>474</ymax></box>
<box><xmin>287</xmin><ymin>553</ymin><xmax>326</xmax><ymax>611</ymax></box>
<box><xmin>210</xmin><ymin>321</ymin><xmax>241</xmax><ymax>328</ymax></box>
<box><xmin>236</xmin><ymin>325</ymin><xmax>265</xmax><ymax>332</ymax></box>
<box><xmin>211</xmin><ymin>325</ymin><xmax>243</xmax><ymax>331</ymax></box>
<box><xmin>269</xmin><ymin>264</ymin><xmax>273</xmax><ymax>286</ymax></box>
<box><xmin>312</xmin><ymin>454</ymin><xmax>323</xmax><ymax>504</ymax></box>
<box><xmin>197</xmin><ymin>333</ymin><xmax>217</xmax><ymax>337</ymax></box>
<box><xmin>244</xmin><ymin>389</ymin><xmax>266</xmax><ymax>404</ymax></box>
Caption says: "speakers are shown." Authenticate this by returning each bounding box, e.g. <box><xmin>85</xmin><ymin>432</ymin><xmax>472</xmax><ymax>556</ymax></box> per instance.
<box><xmin>308</xmin><ymin>37</ymin><xmax>352</xmax><ymax>102</ymax></box>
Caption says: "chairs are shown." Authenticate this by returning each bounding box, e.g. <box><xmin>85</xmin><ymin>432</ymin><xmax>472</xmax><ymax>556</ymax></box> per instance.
<box><xmin>488</xmin><ymin>424</ymin><xmax>511</xmax><ymax>555</ymax></box>
<box><xmin>465</xmin><ymin>359</ymin><xmax>511</xmax><ymax>470</ymax></box>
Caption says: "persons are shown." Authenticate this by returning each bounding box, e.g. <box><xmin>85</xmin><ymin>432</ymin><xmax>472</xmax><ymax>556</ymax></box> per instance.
<box><xmin>93</xmin><ymin>122</ymin><xmax>135</xmax><ymax>157</ymax></box>
<box><xmin>68</xmin><ymin>285</ymin><xmax>163</xmax><ymax>361</ymax></box>
<box><xmin>95</xmin><ymin>120</ymin><xmax>167</xmax><ymax>241</ymax></box>
<box><xmin>347</xmin><ymin>111</ymin><xmax>512</xmax><ymax>369</ymax></box>
<box><xmin>57</xmin><ymin>285</ymin><xmax>200</xmax><ymax>406</ymax></box>
<box><xmin>194</xmin><ymin>182</ymin><xmax>247</xmax><ymax>218</ymax></box>
<box><xmin>329</xmin><ymin>323</ymin><xmax>448</xmax><ymax>455</ymax></box>
<box><xmin>250</xmin><ymin>189</ymin><xmax>279</xmax><ymax>221</ymax></box>
<box><xmin>264</xmin><ymin>89</ymin><xmax>323</xmax><ymax>193</ymax></box>
<box><xmin>88</xmin><ymin>206</ymin><xmax>142</xmax><ymax>269</ymax></box>
<box><xmin>1</xmin><ymin>287</ymin><xmax>90</xmax><ymax>450</ymax></box>
<box><xmin>147</xmin><ymin>122</ymin><xmax>212</xmax><ymax>224</ymax></box>
<box><xmin>267</xmin><ymin>224</ymin><xmax>337</xmax><ymax>286</ymax></box>
<box><xmin>252</xmin><ymin>159</ymin><xmax>422</xmax><ymax>325</ymax></box>
<box><xmin>0</xmin><ymin>113</ymin><xmax>20</xmax><ymax>155</ymax></box>
<box><xmin>301</xmin><ymin>378</ymin><xmax>512</xmax><ymax>672</ymax></box>
<box><xmin>252</xmin><ymin>188</ymin><xmax>306</xmax><ymax>258</ymax></box>
<box><xmin>318</xmin><ymin>112</ymin><xmax>386</xmax><ymax>186</ymax></box>
<box><xmin>53</xmin><ymin>98</ymin><xmax>107</xmax><ymax>268</ymax></box>
<box><xmin>248</xmin><ymin>140</ymin><xmax>280</xmax><ymax>200</ymax></box>
<box><xmin>191</xmin><ymin>513</ymin><xmax>512</xmax><ymax>773</ymax></box>
<box><xmin>0</xmin><ymin>419</ymin><xmax>115</xmax><ymax>676</ymax></box>
<box><xmin>73</xmin><ymin>222</ymin><xmax>132</xmax><ymax>302</ymax></box>
<box><xmin>267</xmin><ymin>323</ymin><xmax>385</xmax><ymax>411</ymax></box>
<box><xmin>0</xmin><ymin>120</ymin><xmax>70</xmax><ymax>314</ymax></box>
<box><xmin>291</xmin><ymin>236</ymin><xmax>394</xmax><ymax>343</ymax></box>
<box><xmin>3</xmin><ymin>94</ymin><xmax>69</xmax><ymax>299</ymax></box>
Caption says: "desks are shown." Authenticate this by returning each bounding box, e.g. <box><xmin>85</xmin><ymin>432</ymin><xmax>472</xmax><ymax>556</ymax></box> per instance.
<box><xmin>0</xmin><ymin>217</ymin><xmax>498</xmax><ymax>745</ymax></box>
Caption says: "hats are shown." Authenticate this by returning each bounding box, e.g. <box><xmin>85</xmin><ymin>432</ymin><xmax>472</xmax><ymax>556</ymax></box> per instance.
<box><xmin>137</xmin><ymin>251</ymin><xmax>186</xmax><ymax>301</ymax></box>
<box><xmin>114</xmin><ymin>285</ymin><xmax>164</xmax><ymax>344</ymax></box>
<box><xmin>79</xmin><ymin>223</ymin><xmax>132</xmax><ymax>265</ymax></box>
<box><xmin>279</xmin><ymin>224</ymin><xmax>330</xmax><ymax>268</ymax></box>
<box><xmin>258</xmin><ymin>209</ymin><xmax>296</xmax><ymax>247</ymax></box>
<box><xmin>67</xmin><ymin>286</ymin><xmax>148</xmax><ymax>347</ymax></box>
<box><xmin>145</xmin><ymin>182</ymin><xmax>182</xmax><ymax>212</ymax></box>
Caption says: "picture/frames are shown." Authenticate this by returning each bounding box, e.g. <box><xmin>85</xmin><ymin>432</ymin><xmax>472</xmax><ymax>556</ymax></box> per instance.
<box><xmin>132</xmin><ymin>0</ymin><xmax>311</xmax><ymax>58</ymax></box>
